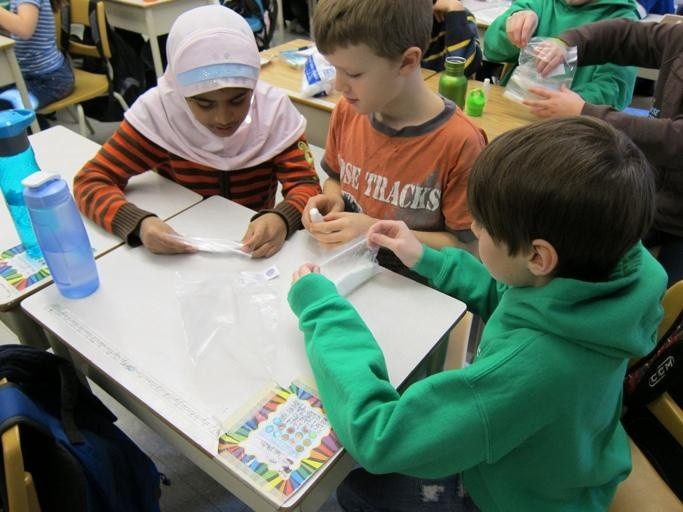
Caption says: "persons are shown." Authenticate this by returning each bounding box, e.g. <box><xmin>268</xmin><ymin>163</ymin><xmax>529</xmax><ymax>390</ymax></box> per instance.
<box><xmin>424</xmin><ymin>1</ymin><xmax>482</xmax><ymax>81</ymax></box>
<box><xmin>287</xmin><ymin>114</ymin><xmax>668</xmax><ymax>512</ymax></box>
<box><xmin>523</xmin><ymin>18</ymin><xmax>683</xmax><ymax>274</ymax></box>
<box><xmin>72</xmin><ymin>7</ymin><xmax>323</xmax><ymax>259</ymax></box>
<box><xmin>302</xmin><ymin>0</ymin><xmax>486</xmax><ymax>263</ymax></box>
<box><xmin>1</xmin><ymin>0</ymin><xmax>74</xmax><ymax>109</ymax></box>
<box><xmin>483</xmin><ymin>1</ymin><xmax>645</xmax><ymax>111</ymax></box>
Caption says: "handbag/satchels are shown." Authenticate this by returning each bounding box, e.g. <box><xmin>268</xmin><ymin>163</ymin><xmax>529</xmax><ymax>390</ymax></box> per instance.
<box><xmin>1</xmin><ymin>344</ymin><xmax>161</xmax><ymax>511</ymax></box>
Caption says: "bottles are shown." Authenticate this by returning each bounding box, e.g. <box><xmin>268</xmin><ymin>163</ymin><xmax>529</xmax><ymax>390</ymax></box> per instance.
<box><xmin>20</xmin><ymin>168</ymin><xmax>100</xmax><ymax>300</ymax></box>
<box><xmin>0</xmin><ymin>107</ymin><xmax>45</xmax><ymax>264</ymax></box>
<box><xmin>439</xmin><ymin>55</ymin><xmax>469</xmax><ymax>113</ymax></box>
